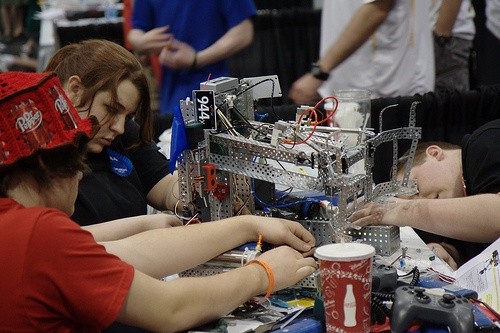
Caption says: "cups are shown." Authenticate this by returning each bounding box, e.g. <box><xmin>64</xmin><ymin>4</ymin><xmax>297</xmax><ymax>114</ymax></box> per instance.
<box><xmin>333</xmin><ymin>88</ymin><xmax>372</xmax><ymax>149</ymax></box>
<box><xmin>313</xmin><ymin>242</ymin><xmax>376</xmax><ymax>333</ymax></box>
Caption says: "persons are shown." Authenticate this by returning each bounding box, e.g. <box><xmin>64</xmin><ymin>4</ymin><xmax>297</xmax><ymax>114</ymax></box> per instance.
<box><xmin>291</xmin><ymin>0</ymin><xmax>500</xmax><ymax>147</ymax></box>
<box><xmin>348</xmin><ymin>136</ymin><xmax>500</xmax><ymax>271</ymax></box>
<box><xmin>0</xmin><ymin>72</ymin><xmax>318</xmax><ymax>333</ymax></box>
<box><xmin>49</xmin><ymin>42</ymin><xmax>181</xmax><ymax>225</ymax></box>
<box><xmin>129</xmin><ymin>1</ymin><xmax>258</xmax><ymax>139</ymax></box>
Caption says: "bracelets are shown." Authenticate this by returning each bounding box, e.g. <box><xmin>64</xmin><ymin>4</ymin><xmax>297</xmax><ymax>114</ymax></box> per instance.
<box><xmin>245</xmin><ymin>258</ymin><xmax>274</xmax><ymax>302</ymax></box>
<box><xmin>191</xmin><ymin>51</ymin><xmax>199</xmax><ymax>68</ymax></box>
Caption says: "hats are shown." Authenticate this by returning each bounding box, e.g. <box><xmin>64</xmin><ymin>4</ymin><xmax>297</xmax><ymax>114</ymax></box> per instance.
<box><xmin>0</xmin><ymin>70</ymin><xmax>101</xmax><ymax>167</ymax></box>
